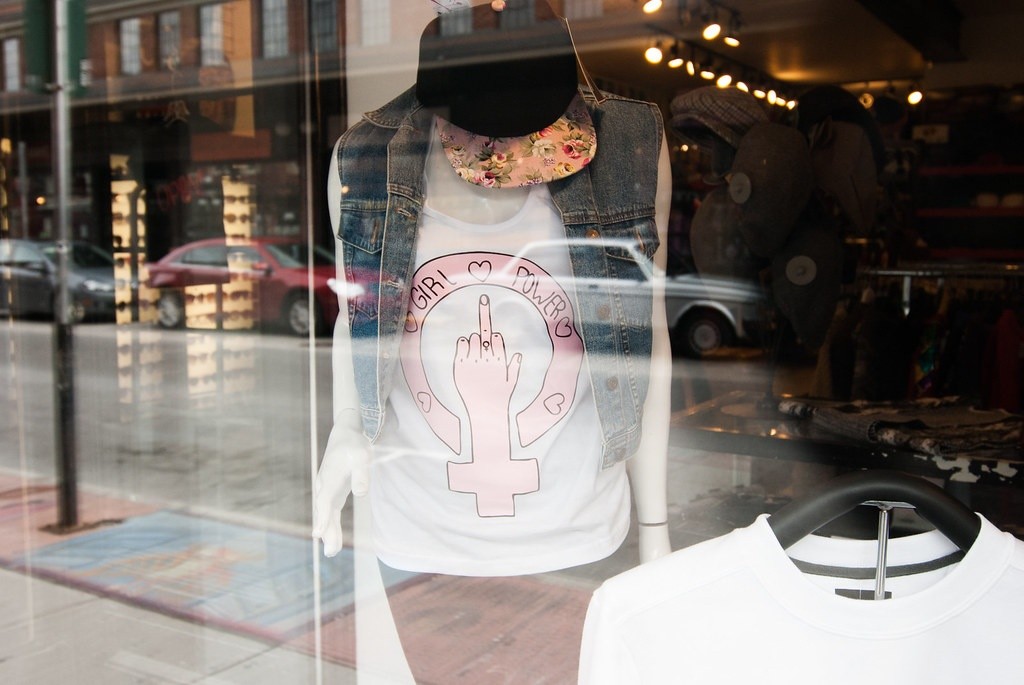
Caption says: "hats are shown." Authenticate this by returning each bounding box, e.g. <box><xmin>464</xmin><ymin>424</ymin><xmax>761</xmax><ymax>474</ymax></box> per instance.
<box><xmin>670</xmin><ymin>86</ymin><xmax>890</xmax><ymax>347</ymax></box>
<box><xmin>415</xmin><ymin>0</ymin><xmax>598</xmax><ymax>190</ymax></box>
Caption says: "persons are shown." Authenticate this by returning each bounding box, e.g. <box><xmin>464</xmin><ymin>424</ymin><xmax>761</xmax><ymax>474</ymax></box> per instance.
<box><xmin>315</xmin><ymin>0</ymin><xmax>673</xmax><ymax>685</ymax></box>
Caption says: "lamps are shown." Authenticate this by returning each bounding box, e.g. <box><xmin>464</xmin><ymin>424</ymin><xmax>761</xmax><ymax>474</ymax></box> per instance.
<box><xmin>906</xmin><ymin>85</ymin><xmax>923</xmax><ymax>107</ymax></box>
<box><xmin>696</xmin><ymin>2</ymin><xmax>722</xmax><ymax>42</ymax></box>
<box><xmin>752</xmin><ymin>71</ymin><xmax>798</xmax><ymax>110</ymax></box>
<box><xmin>736</xmin><ymin>66</ymin><xmax>752</xmax><ymax>94</ymax></box>
<box><xmin>699</xmin><ymin>52</ymin><xmax>716</xmax><ymax>81</ymax></box>
<box><xmin>685</xmin><ymin>43</ymin><xmax>701</xmax><ymax>77</ymax></box>
<box><xmin>715</xmin><ymin>61</ymin><xmax>733</xmax><ymax>90</ymax></box>
<box><xmin>642</xmin><ymin>30</ymin><xmax>666</xmax><ymax>67</ymax></box>
<box><xmin>723</xmin><ymin>13</ymin><xmax>748</xmax><ymax>51</ymax></box>
<box><xmin>633</xmin><ymin>0</ymin><xmax>666</xmax><ymax>15</ymax></box>
<box><xmin>665</xmin><ymin>38</ymin><xmax>686</xmax><ymax>70</ymax></box>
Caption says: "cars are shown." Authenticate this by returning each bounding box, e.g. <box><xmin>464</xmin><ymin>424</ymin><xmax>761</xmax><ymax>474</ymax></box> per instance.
<box><xmin>138</xmin><ymin>236</ymin><xmax>409</xmax><ymax>336</ymax></box>
<box><xmin>438</xmin><ymin>238</ymin><xmax>777</xmax><ymax>360</ymax></box>
<box><xmin>0</xmin><ymin>237</ymin><xmax>138</xmax><ymax>323</ymax></box>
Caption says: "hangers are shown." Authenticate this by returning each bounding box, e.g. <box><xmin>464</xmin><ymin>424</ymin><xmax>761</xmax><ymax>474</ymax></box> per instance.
<box><xmin>769</xmin><ymin>424</ymin><xmax>980</xmax><ymax>577</ymax></box>
<box><xmin>856</xmin><ymin>264</ymin><xmax>985</xmax><ymax>315</ymax></box>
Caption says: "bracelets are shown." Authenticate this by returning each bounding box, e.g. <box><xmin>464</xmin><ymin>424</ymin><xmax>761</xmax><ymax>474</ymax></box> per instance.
<box><xmin>638</xmin><ymin>520</ymin><xmax>668</xmax><ymax>526</ymax></box>
